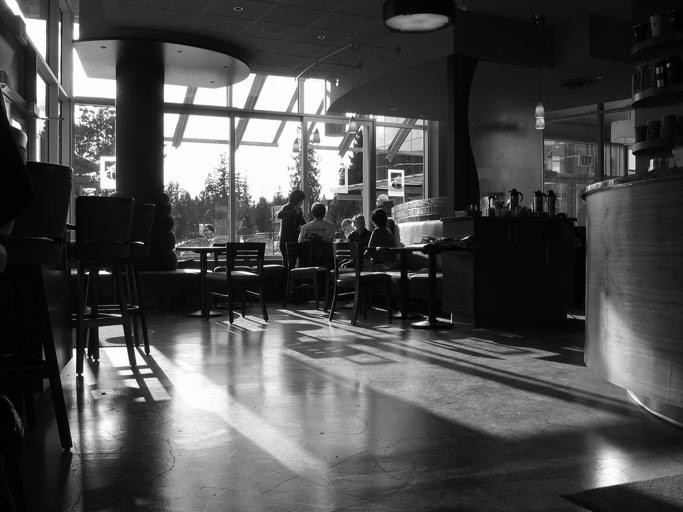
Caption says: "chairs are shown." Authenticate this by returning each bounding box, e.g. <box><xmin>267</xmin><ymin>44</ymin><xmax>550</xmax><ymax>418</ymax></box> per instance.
<box><xmin>0</xmin><ymin>157</ymin><xmax>159</xmax><ymax>451</ymax></box>
<box><xmin>159</xmin><ymin>238</ymin><xmax>475</xmax><ymax>330</ymax></box>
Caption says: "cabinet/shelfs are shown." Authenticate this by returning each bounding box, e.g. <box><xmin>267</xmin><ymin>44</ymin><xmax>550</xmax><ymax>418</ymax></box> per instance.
<box><xmin>439</xmin><ymin>215</ymin><xmax>577</xmax><ymax>330</ymax></box>
<box><xmin>629</xmin><ymin>30</ymin><xmax>682</xmax><ymax>156</ymax></box>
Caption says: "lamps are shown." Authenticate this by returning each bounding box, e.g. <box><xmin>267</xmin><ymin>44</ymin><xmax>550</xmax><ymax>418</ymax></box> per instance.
<box><xmin>533</xmin><ymin>83</ymin><xmax>545</xmax><ymax>129</ymax></box>
<box><xmin>381</xmin><ymin>0</ymin><xmax>458</xmax><ymax>34</ymax></box>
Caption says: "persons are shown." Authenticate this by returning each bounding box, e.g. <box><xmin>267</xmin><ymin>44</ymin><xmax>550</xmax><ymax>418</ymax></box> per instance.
<box><xmin>277</xmin><ymin>188</ymin><xmax>306</xmax><ymax>268</ymax></box>
<box><xmin>294</xmin><ymin>201</ymin><xmax>336</xmax><ymax>267</ymax></box>
<box><xmin>335</xmin><ymin>217</ymin><xmax>355</xmax><ymax>241</ymax></box>
<box><xmin>360</xmin><ymin>208</ymin><xmax>394</xmax><ymax>272</ymax></box>
<box><xmin>341</xmin><ymin>213</ymin><xmax>371</xmax><ymax>268</ymax></box>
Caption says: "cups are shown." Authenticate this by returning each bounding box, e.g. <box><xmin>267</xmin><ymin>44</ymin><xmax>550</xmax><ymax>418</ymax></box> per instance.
<box><xmin>666</xmin><ymin>156</ymin><xmax>676</xmax><ymax>169</ymax></box>
<box><xmin>632</xmin><ymin>9</ymin><xmax>679</xmax><ymax>43</ymax></box>
<box><xmin>533</xmin><ymin>190</ymin><xmax>546</xmax><ymax>216</ymax></box>
<box><xmin>634</xmin><ymin>124</ymin><xmax>647</xmax><ymax>141</ymax></box>
<box><xmin>655</xmin><ymin>62</ymin><xmax>670</xmax><ymax>88</ymax></box>
<box><xmin>509</xmin><ymin>188</ymin><xmax>523</xmax><ymax>207</ymax></box>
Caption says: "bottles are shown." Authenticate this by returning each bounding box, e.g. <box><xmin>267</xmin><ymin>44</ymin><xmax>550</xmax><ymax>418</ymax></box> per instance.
<box><xmin>546</xmin><ymin>189</ymin><xmax>556</xmax><ymax>218</ymax></box>
<box><xmin>647</xmin><ymin>114</ymin><xmax>683</xmax><ymax>140</ymax></box>
